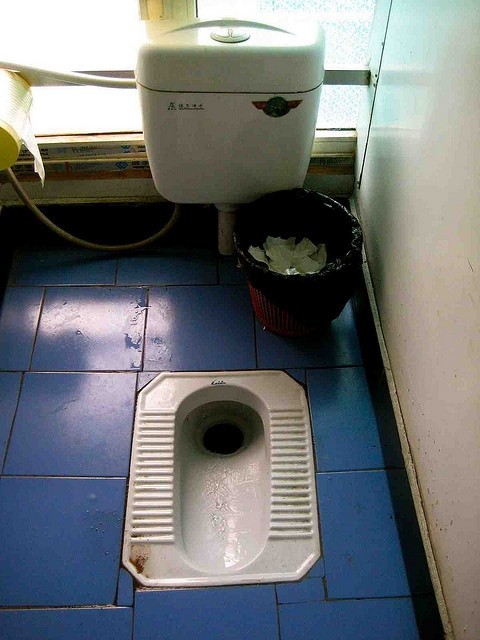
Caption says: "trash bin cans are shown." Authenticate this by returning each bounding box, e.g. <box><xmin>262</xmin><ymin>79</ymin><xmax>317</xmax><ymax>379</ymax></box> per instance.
<box><xmin>233</xmin><ymin>188</ymin><xmax>362</xmax><ymax>335</ymax></box>
